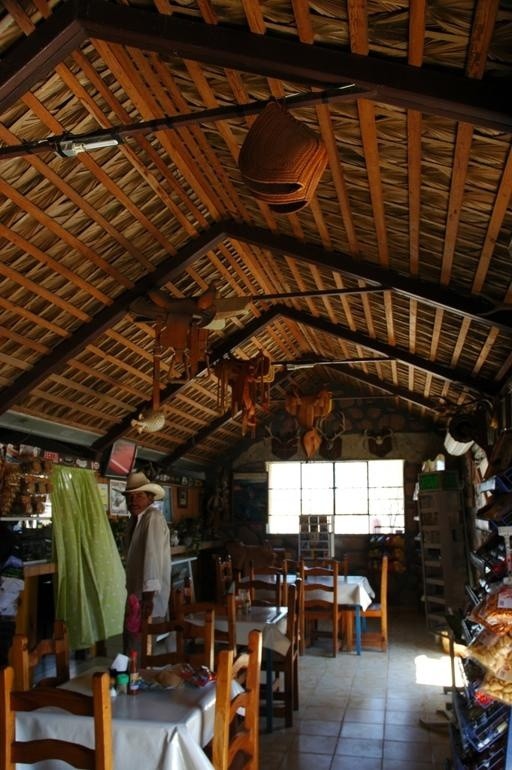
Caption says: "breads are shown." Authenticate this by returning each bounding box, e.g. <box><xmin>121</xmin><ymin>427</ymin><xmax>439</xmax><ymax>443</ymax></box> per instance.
<box><xmin>157</xmin><ymin>669</ymin><xmax>181</xmax><ymax>688</ymax></box>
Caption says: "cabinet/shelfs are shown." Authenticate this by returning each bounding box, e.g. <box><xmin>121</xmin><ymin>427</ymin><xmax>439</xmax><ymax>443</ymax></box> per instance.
<box><xmin>414</xmin><ymin>468</ymin><xmax>511</xmax><ymax>770</ymax></box>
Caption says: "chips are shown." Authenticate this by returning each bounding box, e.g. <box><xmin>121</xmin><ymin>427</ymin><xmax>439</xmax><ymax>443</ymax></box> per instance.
<box><xmin>480</xmin><ymin>675</ymin><xmax>512</xmax><ymax>704</ymax></box>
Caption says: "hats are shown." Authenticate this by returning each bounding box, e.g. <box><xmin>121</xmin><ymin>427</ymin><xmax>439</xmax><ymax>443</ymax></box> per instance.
<box><xmin>112</xmin><ymin>472</ymin><xmax>166</xmax><ymax>501</ymax></box>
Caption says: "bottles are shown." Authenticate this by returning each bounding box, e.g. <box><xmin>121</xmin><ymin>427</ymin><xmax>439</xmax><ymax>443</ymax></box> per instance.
<box><xmin>129</xmin><ymin>651</ymin><xmax>141</xmax><ymax>695</ymax></box>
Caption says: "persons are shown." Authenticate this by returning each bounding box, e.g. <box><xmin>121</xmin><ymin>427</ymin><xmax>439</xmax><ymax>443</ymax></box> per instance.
<box><xmin>114</xmin><ymin>492</ymin><xmax>126</xmax><ymax>507</ymax></box>
<box><xmin>111</xmin><ymin>472</ymin><xmax>174</xmax><ymax>669</ymax></box>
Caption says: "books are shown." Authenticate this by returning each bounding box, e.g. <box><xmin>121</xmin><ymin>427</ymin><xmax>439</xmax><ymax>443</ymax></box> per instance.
<box><xmin>298</xmin><ymin>515</ymin><xmax>331</xmax><ymax>570</ymax></box>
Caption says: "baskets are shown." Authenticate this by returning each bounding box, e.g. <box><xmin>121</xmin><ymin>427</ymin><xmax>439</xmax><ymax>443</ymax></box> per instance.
<box><xmin>448</xmin><ymin>680</ymin><xmax>510</xmax><ymax>770</ymax></box>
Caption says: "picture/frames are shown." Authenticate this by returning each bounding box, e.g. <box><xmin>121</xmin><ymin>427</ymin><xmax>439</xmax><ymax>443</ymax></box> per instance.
<box><xmin>107</xmin><ymin>478</ymin><xmax>191</xmax><ymax>524</ymax></box>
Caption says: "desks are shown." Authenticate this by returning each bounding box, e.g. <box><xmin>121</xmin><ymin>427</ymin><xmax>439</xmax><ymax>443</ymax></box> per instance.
<box><xmin>15</xmin><ymin>657</ymin><xmax>248</xmax><ymax>768</ymax></box>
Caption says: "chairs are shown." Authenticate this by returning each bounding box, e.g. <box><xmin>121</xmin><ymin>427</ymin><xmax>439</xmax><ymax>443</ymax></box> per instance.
<box><xmin>1</xmin><ymin>666</ymin><xmax>112</xmax><ymax>770</ymax></box>
<box><xmin>10</xmin><ymin>619</ymin><xmax>71</xmax><ymax>688</ymax></box>
<box><xmin>139</xmin><ymin>609</ymin><xmax>216</xmax><ymax>671</ymax></box>
<box><xmin>201</xmin><ymin>628</ymin><xmax>264</xmax><ymax>768</ymax></box>
<box><xmin>259</xmin><ymin>578</ymin><xmax>305</xmax><ymax>732</ymax></box>
<box><xmin>0</xmin><ymin>547</ymin><xmax>392</xmax><ymax>650</ymax></box>
